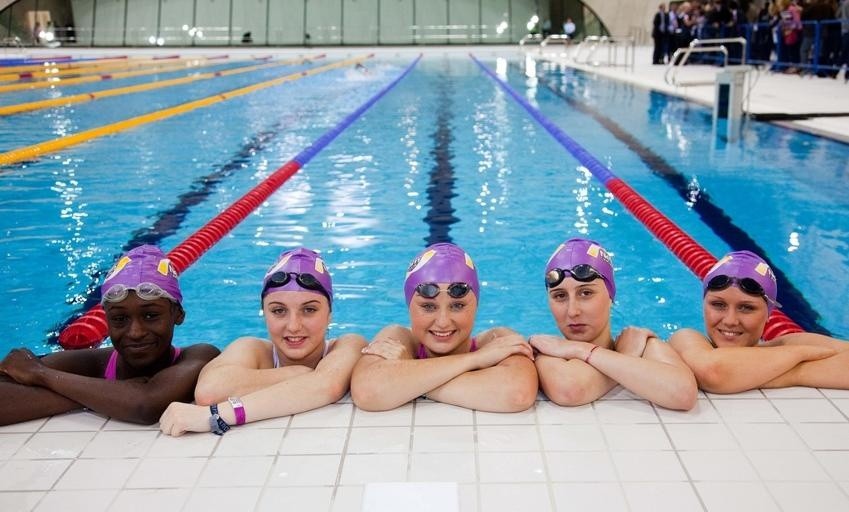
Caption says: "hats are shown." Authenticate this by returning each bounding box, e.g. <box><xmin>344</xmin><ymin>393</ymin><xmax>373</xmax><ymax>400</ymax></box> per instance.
<box><xmin>101</xmin><ymin>245</ymin><xmax>182</xmax><ymax>303</ymax></box>
<box><xmin>262</xmin><ymin>248</ymin><xmax>333</xmax><ymax>301</ymax></box>
<box><xmin>702</xmin><ymin>250</ymin><xmax>777</xmax><ymax>316</ymax></box>
<box><xmin>404</xmin><ymin>241</ymin><xmax>479</xmax><ymax>305</ymax></box>
<box><xmin>544</xmin><ymin>237</ymin><xmax>616</xmax><ymax>303</ymax></box>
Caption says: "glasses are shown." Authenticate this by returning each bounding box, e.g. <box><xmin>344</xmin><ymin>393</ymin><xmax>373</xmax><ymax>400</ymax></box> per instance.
<box><xmin>413</xmin><ymin>282</ymin><xmax>470</xmax><ymax>299</ymax></box>
<box><xmin>261</xmin><ymin>272</ymin><xmax>331</xmax><ymax>309</ymax></box>
<box><xmin>543</xmin><ymin>264</ymin><xmax>609</xmax><ymax>288</ymax></box>
<box><xmin>101</xmin><ymin>283</ymin><xmax>178</xmax><ymax>305</ymax></box>
<box><xmin>705</xmin><ymin>274</ymin><xmax>766</xmax><ymax>299</ymax></box>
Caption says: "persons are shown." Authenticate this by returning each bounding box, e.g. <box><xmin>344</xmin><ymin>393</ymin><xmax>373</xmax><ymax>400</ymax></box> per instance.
<box><xmin>525</xmin><ymin>238</ymin><xmax>699</xmax><ymax>413</ymax></box>
<box><xmin>348</xmin><ymin>242</ymin><xmax>541</xmax><ymax>416</ymax></box>
<box><xmin>562</xmin><ymin>17</ymin><xmax>576</xmax><ymax>40</ymax></box>
<box><xmin>44</xmin><ymin>21</ymin><xmax>55</xmax><ymax>44</ymax></box>
<box><xmin>665</xmin><ymin>247</ymin><xmax>849</xmax><ymax>396</ymax></box>
<box><xmin>0</xmin><ymin>242</ymin><xmax>222</xmax><ymax>429</ymax></box>
<box><xmin>651</xmin><ymin>0</ymin><xmax>849</xmax><ymax>83</ymax></box>
<box><xmin>153</xmin><ymin>248</ymin><xmax>367</xmax><ymax>438</ymax></box>
<box><xmin>32</xmin><ymin>22</ymin><xmax>42</xmax><ymax>44</ymax></box>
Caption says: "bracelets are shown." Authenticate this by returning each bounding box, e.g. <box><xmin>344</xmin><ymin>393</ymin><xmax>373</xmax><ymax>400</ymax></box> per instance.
<box><xmin>208</xmin><ymin>402</ymin><xmax>230</xmax><ymax>436</ymax></box>
<box><xmin>583</xmin><ymin>343</ymin><xmax>601</xmax><ymax>364</ymax></box>
<box><xmin>228</xmin><ymin>395</ymin><xmax>246</xmax><ymax>426</ymax></box>
